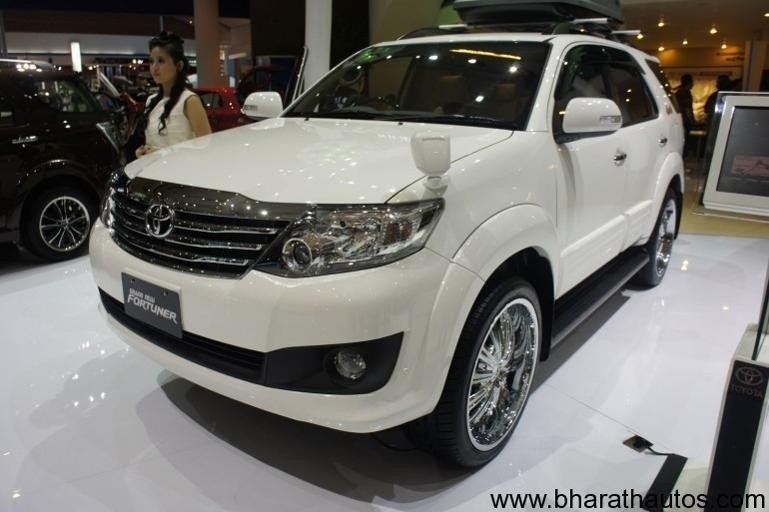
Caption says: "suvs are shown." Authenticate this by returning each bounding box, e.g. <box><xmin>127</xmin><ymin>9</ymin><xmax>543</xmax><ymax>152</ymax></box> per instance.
<box><xmin>1</xmin><ymin>59</ymin><xmax>117</xmax><ymax>263</ymax></box>
<box><xmin>80</xmin><ymin>1</ymin><xmax>689</xmax><ymax>471</ymax></box>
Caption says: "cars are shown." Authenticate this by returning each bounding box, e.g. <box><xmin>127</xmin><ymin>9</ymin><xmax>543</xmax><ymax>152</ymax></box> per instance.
<box><xmin>119</xmin><ymin>64</ymin><xmax>277</xmax><ymax>145</ymax></box>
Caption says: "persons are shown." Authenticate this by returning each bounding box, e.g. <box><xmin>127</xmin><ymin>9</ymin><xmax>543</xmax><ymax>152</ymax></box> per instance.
<box><xmin>674</xmin><ymin>74</ymin><xmax>696</xmax><ymax>158</ymax></box>
<box><xmin>704</xmin><ymin>75</ymin><xmax>732</xmax><ymax>115</ymax></box>
<box><xmin>135</xmin><ymin>32</ymin><xmax>213</xmax><ymax>158</ymax></box>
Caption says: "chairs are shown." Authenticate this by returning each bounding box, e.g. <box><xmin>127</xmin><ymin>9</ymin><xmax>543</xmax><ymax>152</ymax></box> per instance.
<box><xmin>487</xmin><ymin>82</ymin><xmax>520</xmax><ymax>122</ymax></box>
<box><xmin>431</xmin><ymin>74</ymin><xmax>471</xmax><ymax>117</ymax></box>
